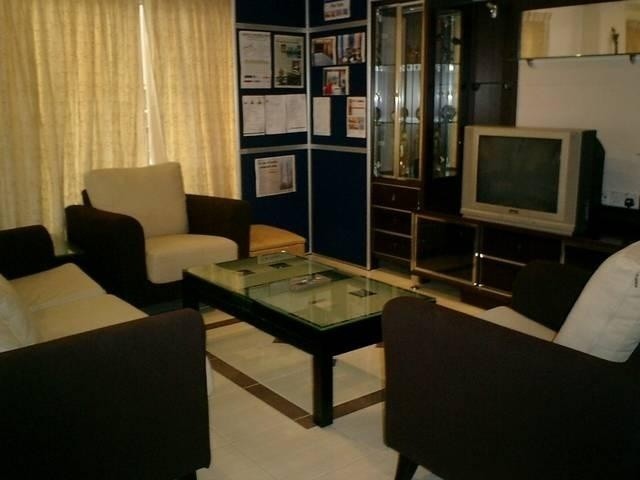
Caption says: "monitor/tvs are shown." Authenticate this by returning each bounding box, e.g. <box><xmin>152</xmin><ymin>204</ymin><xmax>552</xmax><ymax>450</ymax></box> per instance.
<box><xmin>461</xmin><ymin>125</ymin><xmax>605</xmax><ymax>236</ymax></box>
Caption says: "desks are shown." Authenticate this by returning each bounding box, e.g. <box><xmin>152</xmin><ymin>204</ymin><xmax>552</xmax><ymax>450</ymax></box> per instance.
<box><xmin>185</xmin><ymin>252</ymin><xmax>436</xmax><ymax>428</ymax></box>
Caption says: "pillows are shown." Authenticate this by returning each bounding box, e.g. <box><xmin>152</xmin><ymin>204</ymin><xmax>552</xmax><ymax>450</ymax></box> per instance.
<box><xmin>553</xmin><ymin>239</ymin><xmax>638</xmax><ymax>364</ymax></box>
<box><xmin>512</xmin><ymin>246</ymin><xmax>583</xmax><ymax>330</ymax></box>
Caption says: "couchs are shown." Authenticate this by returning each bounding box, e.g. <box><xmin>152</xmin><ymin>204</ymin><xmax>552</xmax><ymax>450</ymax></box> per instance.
<box><xmin>1</xmin><ymin>224</ymin><xmax>211</xmax><ymax>479</ymax></box>
<box><xmin>383</xmin><ymin>261</ymin><xmax>640</xmax><ymax>480</ymax></box>
<box><xmin>66</xmin><ymin>161</ymin><xmax>256</xmax><ymax>308</ymax></box>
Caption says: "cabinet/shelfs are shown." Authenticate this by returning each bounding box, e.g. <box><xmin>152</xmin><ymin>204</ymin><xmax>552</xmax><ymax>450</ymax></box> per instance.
<box><xmin>372</xmin><ymin>178</ymin><xmax>415</xmax><ymax>273</ymax></box>
<box><xmin>563</xmin><ymin>239</ymin><xmax>628</xmax><ymax>280</ymax></box>
<box><xmin>369</xmin><ymin>0</ymin><xmax>464</xmax><ymax>185</ymax></box>
<box><xmin>416</xmin><ymin>215</ymin><xmax>565</xmax><ymax>300</ymax></box>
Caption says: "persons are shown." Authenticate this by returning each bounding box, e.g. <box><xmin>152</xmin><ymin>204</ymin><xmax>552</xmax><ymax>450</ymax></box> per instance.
<box><xmin>324</xmin><ymin>75</ymin><xmax>337</xmax><ymax>95</ymax></box>
<box><xmin>340</xmin><ymin>77</ymin><xmax>346</xmax><ymax>93</ymax></box>
<box><xmin>339</xmin><ymin>47</ymin><xmax>364</xmax><ymax>63</ymax></box>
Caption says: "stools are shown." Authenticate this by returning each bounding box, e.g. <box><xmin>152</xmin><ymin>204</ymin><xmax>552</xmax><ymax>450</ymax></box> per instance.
<box><xmin>249</xmin><ymin>224</ymin><xmax>306</xmax><ymax>257</ymax></box>
